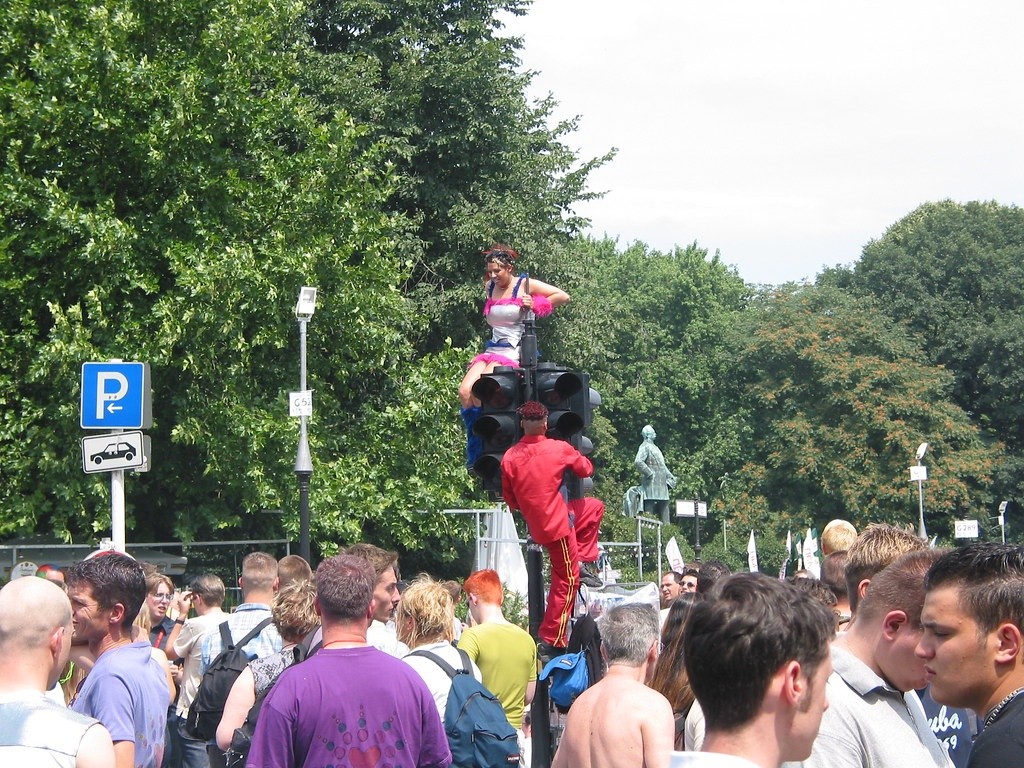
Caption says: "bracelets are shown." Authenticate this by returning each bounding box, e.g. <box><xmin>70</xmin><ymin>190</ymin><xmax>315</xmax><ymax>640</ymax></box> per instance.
<box><xmin>175</xmin><ymin>618</ymin><xmax>184</xmax><ymax>625</ymax></box>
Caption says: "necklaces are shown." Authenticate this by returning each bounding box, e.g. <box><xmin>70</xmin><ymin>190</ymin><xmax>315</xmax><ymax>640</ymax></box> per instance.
<box><xmin>970</xmin><ymin>686</ymin><xmax>1024</xmax><ymax>744</ymax></box>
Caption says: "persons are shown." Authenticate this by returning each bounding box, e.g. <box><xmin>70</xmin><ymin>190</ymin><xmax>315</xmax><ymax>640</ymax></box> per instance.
<box><xmin>457</xmin><ymin>248</ymin><xmax>570</xmax><ymax>477</ymax></box>
<box><xmin>0</xmin><ymin>519</ymin><xmax>1024</xmax><ymax>768</ymax></box>
<box><xmin>633</xmin><ymin>425</ymin><xmax>677</xmax><ymax>524</ymax></box>
<box><xmin>500</xmin><ymin>401</ymin><xmax>605</xmax><ymax>663</ymax></box>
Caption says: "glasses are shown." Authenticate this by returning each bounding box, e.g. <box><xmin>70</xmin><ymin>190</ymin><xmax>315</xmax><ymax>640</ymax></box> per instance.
<box><xmin>678</xmin><ymin>579</ymin><xmax>697</xmax><ymax>587</ymax></box>
<box><xmin>486</xmin><ymin>252</ymin><xmax>509</xmax><ymax>261</ymax></box>
<box><xmin>149</xmin><ymin>591</ymin><xmax>174</xmax><ymax>601</ymax></box>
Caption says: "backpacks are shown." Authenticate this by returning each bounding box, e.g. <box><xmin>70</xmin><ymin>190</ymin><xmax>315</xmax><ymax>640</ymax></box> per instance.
<box><xmin>186</xmin><ymin>614</ymin><xmax>275</xmax><ymax>741</ymax></box>
<box><xmin>402</xmin><ymin>650</ymin><xmax>522</xmax><ymax>768</ymax></box>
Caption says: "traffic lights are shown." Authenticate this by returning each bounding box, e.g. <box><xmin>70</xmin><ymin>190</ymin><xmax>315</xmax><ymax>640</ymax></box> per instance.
<box><xmin>535</xmin><ymin>367</ymin><xmax>601</xmax><ymax>498</ymax></box>
<box><xmin>470</xmin><ymin>370</ymin><xmax>524</xmax><ymax>495</ymax></box>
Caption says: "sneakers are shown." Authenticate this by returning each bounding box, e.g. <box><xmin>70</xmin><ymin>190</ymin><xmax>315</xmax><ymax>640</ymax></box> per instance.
<box><xmin>537</xmin><ymin>643</ymin><xmax>563</xmax><ymax>657</ymax></box>
<box><xmin>578</xmin><ymin>563</ymin><xmax>603</xmax><ymax>587</ymax></box>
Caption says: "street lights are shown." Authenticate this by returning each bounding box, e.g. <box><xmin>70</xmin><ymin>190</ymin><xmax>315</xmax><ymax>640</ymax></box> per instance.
<box><xmin>294</xmin><ymin>286</ymin><xmax>318</xmax><ymax>565</ymax></box>
<box><xmin>915</xmin><ymin>442</ymin><xmax>928</xmax><ymax>540</ymax></box>
<box><xmin>999</xmin><ymin>500</ymin><xmax>1008</xmax><ymax>543</ymax></box>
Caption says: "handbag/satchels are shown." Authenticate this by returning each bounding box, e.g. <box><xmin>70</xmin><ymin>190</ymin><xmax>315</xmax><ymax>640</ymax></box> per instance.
<box><xmin>540</xmin><ymin>651</ymin><xmax>588</xmax><ymax>708</ymax></box>
